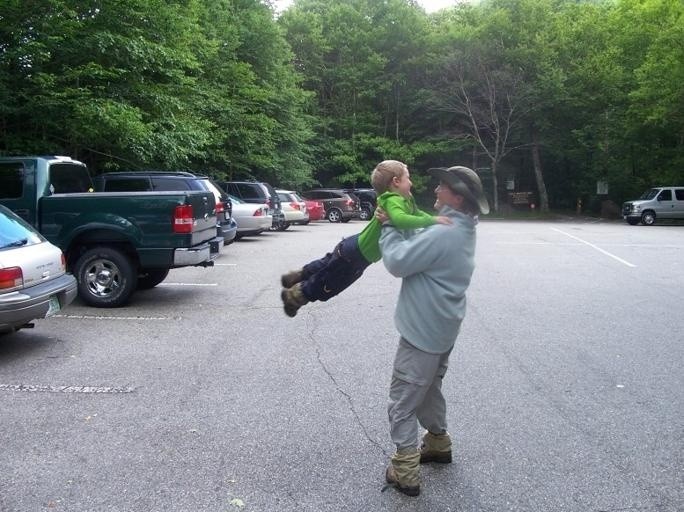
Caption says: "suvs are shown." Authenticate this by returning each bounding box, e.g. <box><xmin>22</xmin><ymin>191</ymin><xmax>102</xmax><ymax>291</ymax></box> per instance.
<box><xmin>216</xmin><ymin>180</ymin><xmax>285</xmax><ymax>236</ymax></box>
<box><xmin>91</xmin><ymin>169</ymin><xmax>237</xmax><ymax>244</ymax></box>
<box><xmin>300</xmin><ymin>188</ymin><xmax>379</xmax><ymax>223</ymax></box>
<box><xmin>620</xmin><ymin>185</ymin><xmax>684</xmax><ymax>227</ymax></box>
<box><xmin>272</xmin><ymin>188</ymin><xmax>310</xmax><ymax>232</ymax></box>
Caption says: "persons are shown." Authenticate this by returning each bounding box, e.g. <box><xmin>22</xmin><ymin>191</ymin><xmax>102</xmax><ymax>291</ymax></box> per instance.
<box><xmin>281</xmin><ymin>159</ymin><xmax>454</xmax><ymax>318</ymax></box>
<box><xmin>375</xmin><ymin>165</ymin><xmax>484</xmax><ymax>497</ymax></box>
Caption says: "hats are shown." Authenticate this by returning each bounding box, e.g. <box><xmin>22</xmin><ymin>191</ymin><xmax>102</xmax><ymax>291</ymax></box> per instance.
<box><xmin>426</xmin><ymin>165</ymin><xmax>491</xmax><ymax>215</ymax></box>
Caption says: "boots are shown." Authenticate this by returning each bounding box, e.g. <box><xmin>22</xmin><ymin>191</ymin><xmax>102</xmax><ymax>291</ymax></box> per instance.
<box><xmin>282</xmin><ymin>270</ymin><xmax>308</xmax><ymax>317</ymax></box>
<box><xmin>417</xmin><ymin>429</ymin><xmax>452</xmax><ymax>463</ymax></box>
<box><xmin>386</xmin><ymin>449</ymin><xmax>420</xmax><ymax>496</ymax></box>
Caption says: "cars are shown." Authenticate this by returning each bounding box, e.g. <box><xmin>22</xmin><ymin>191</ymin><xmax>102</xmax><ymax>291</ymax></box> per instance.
<box><xmin>1</xmin><ymin>204</ymin><xmax>82</xmax><ymax>338</ymax></box>
<box><xmin>227</xmin><ymin>193</ymin><xmax>272</xmax><ymax>239</ymax></box>
<box><xmin>301</xmin><ymin>196</ymin><xmax>325</xmax><ymax>226</ymax></box>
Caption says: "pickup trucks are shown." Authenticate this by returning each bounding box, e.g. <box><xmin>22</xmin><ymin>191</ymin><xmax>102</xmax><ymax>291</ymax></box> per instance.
<box><xmin>1</xmin><ymin>155</ymin><xmax>227</xmax><ymax>309</ymax></box>
